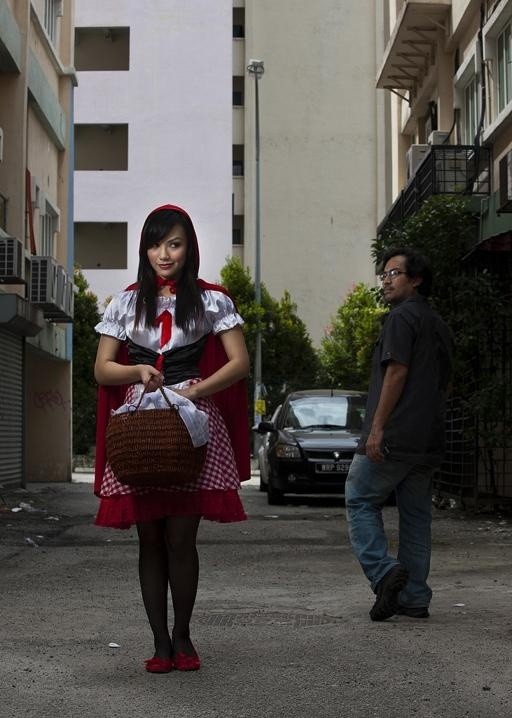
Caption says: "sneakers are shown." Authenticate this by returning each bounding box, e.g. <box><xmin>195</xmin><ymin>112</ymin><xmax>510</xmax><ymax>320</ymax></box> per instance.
<box><xmin>368</xmin><ymin>565</ymin><xmax>431</xmax><ymax>622</ymax></box>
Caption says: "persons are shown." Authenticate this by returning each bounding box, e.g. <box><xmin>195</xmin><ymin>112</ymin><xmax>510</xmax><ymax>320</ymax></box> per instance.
<box><xmin>343</xmin><ymin>247</ymin><xmax>456</xmax><ymax>622</ymax></box>
<box><xmin>93</xmin><ymin>203</ymin><xmax>253</xmax><ymax>674</ymax></box>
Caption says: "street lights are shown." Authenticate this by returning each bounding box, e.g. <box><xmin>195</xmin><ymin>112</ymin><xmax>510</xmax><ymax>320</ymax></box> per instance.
<box><xmin>244</xmin><ymin>57</ymin><xmax>267</xmax><ymax>469</ymax></box>
<box><xmin>254</xmin><ymin>388</ymin><xmax>401</xmax><ymax>507</ymax></box>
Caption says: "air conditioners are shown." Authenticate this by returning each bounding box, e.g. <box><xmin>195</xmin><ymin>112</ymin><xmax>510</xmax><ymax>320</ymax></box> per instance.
<box><xmin>0</xmin><ymin>235</ymin><xmax>76</xmax><ymax>324</ymax></box>
<box><xmin>402</xmin><ymin>129</ymin><xmax>467</xmax><ymax>195</ymax></box>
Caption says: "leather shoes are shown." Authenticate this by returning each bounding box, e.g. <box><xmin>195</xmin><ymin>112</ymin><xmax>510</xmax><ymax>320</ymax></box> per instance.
<box><xmin>146</xmin><ymin>634</ymin><xmax>201</xmax><ymax>672</ymax></box>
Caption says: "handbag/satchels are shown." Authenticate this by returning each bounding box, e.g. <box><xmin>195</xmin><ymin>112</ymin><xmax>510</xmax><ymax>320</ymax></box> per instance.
<box><xmin>106</xmin><ymin>374</ymin><xmax>208</xmax><ymax>485</ymax></box>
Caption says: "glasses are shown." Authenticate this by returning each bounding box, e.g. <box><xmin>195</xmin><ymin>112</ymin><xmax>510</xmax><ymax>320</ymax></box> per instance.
<box><xmin>381</xmin><ymin>268</ymin><xmax>406</xmax><ymax>279</ymax></box>
